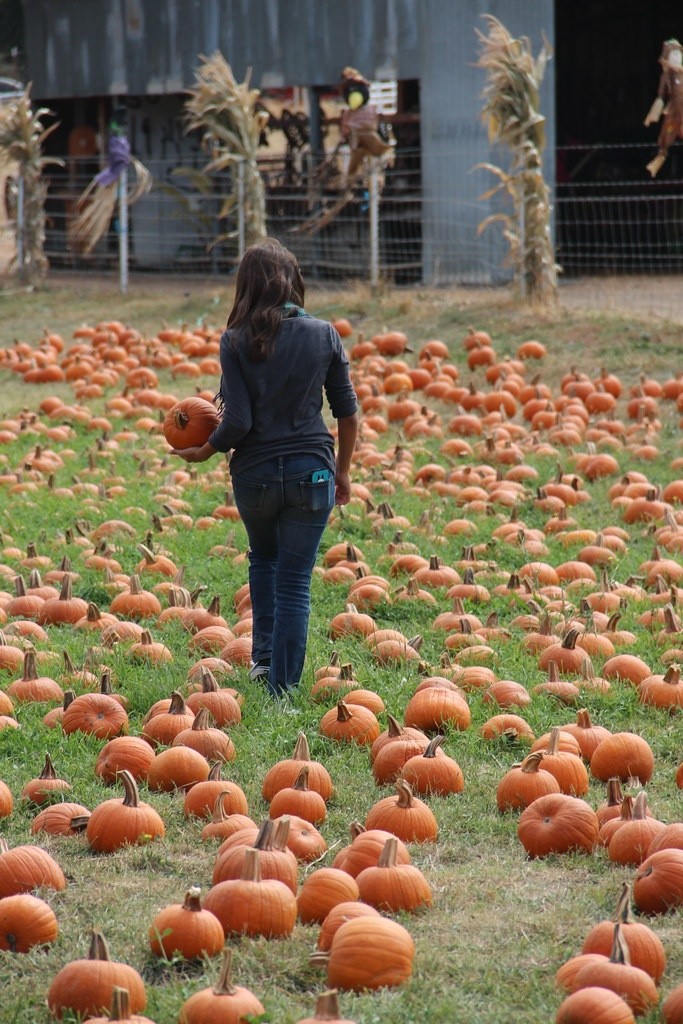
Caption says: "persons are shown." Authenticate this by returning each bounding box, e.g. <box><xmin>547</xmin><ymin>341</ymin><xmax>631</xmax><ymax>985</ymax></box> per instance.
<box><xmin>166</xmin><ymin>236</ymin><xmax>360</xmax><ymax>705</ymax></box>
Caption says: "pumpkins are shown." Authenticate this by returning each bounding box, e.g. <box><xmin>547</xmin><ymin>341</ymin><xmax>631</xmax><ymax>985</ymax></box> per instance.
<box><xmin>0</xmin><ymin>320</ymin><xmax>683</xmax><ymax>1024</ymax></box>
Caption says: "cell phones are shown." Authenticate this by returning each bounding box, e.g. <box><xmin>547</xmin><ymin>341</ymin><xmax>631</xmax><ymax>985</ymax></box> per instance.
<box><xmin>310</xmin><ymin>469</ymin><xmax>328</xmax><ymax>483</ymax></box>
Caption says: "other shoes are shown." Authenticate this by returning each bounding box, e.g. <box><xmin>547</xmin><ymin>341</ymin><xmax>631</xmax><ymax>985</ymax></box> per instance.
<box><xmin>251</xmin><ymin>656</ymin><xmax>272</xmax><ymax>680</ymax></box>
